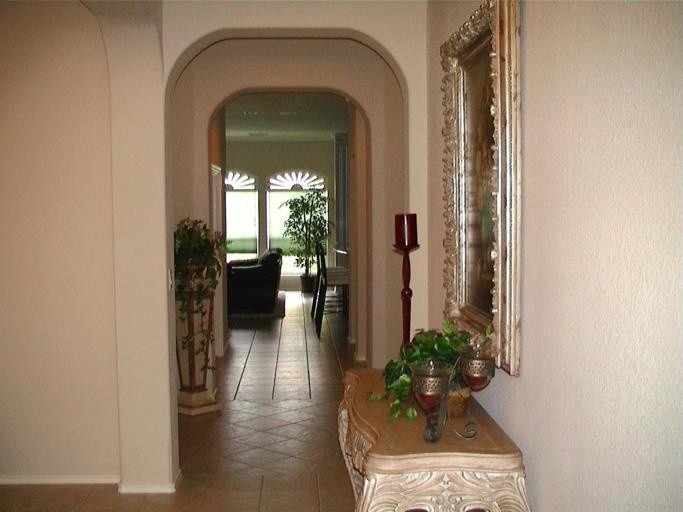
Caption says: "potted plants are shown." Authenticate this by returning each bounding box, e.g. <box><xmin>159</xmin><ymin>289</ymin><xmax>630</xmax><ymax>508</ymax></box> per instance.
<box><xmin>278</xmin><ymin>184</ymin><xmax>336</xmax><ymax>293</ymax></box>
<box><xmin>367</xmin><ymin>315</ymin><xmax>496</xmax><ymax>421</ymax></box>
<box><xmin>172</xmin><ymin>217</ymin><xmax>233</xmax><ymax>373</ymax></box>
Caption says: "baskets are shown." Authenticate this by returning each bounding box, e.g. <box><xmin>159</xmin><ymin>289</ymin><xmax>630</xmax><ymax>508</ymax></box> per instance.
<box><xmin>446</xmin><ymin>395</ymin><xmax>470</xmax><ymax>416</ymax></box>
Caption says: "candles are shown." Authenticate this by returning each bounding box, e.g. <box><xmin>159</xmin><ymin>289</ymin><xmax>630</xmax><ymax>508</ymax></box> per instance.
<box><xmin>395</xmin><ymin>213</ymin><xmax>418</xmax><ymax>245</ymax></box>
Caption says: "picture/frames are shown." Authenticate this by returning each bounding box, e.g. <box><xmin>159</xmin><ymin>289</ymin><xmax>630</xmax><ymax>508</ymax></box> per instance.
<box><xmin>440</xmin><ymin>0</ymin><xmax>522</xmax><ymax>377</ymax></box>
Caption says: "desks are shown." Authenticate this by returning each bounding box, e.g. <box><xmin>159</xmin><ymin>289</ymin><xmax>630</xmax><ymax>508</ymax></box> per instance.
<box><xmin>338</xmin><ymin>367</ymin><xmax>534</xmax><ymax>512</ymax></box>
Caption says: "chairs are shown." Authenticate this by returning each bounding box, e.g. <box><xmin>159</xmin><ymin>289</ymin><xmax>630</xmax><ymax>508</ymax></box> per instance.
<box><xmin>226</xmin><ymin>248</ymin><xmax>283</xmax><ymax>312</ymax></box>
<box><xmin>311</xmin><ymin>244</ymin><xmax>350</xmax><ymax>338</ymax></box>
